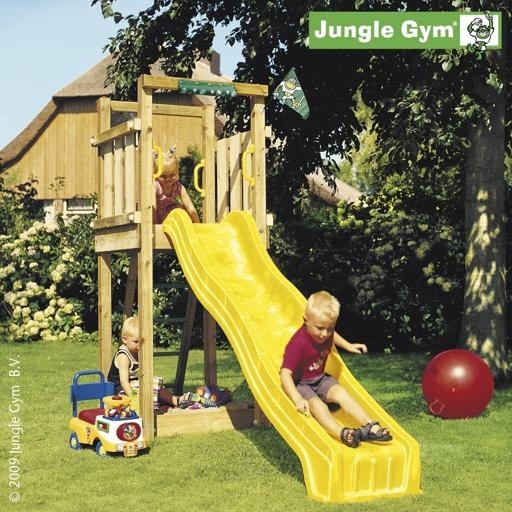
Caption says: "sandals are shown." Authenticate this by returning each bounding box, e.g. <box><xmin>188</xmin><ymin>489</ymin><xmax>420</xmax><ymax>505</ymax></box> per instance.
<box><xmin>177</xmin><ymin>393</ymin><xmax>193</xmax><ymax>409</ymax></box>
<box><xmin>340</xmin><ymin>420</ymin><xmax>392</xmax><ymax>448</ymax></box>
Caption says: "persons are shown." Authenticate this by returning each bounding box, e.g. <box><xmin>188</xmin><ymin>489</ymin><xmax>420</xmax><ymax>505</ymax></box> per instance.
<box><xmin>152</xmin><ymin>145</ymin><xmax>200</xmax><ymax>224</ymax></box>
<box><xmin>68</xmin><ymin>370</ymin><xmax>147</xmax><ymax>457</ymax></box>
<box><xmin>281</xmin><ymin>289</ymin><xmax>390</xmax><ymax>445</ymax></box>
<box><xmin>106</xmin><ymin>316</ymin><xmax>193</xmax><ymax>410</ymax></box>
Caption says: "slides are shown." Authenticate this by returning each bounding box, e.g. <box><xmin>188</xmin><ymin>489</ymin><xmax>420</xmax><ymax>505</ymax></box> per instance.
<box><xmin>162</xmin><ymin>209</ymin><xmax>422</xmax><ymax>504</ymax></box>
